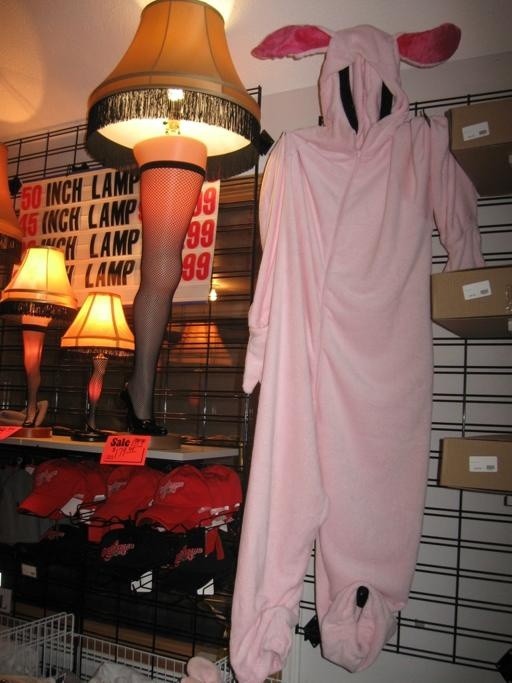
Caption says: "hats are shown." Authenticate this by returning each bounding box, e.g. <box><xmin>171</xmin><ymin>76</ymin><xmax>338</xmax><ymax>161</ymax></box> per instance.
<box><xmin>16</xmin><ymin>458</ymin><xmax>242</xmax><ymax>533</ymax></box>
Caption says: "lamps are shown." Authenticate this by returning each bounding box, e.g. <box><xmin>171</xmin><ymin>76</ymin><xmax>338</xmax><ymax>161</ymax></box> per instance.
<box><xmin>0</xmin><ymin>143</ymin><xmax>27</xmax><ymax>248</ymax></box>
<box><xmin>2</xmin><ymin>238</ymin><xmax>85</xmax><ymax>438</ymax></box>
<box><xmin>82</xmin><ymin>0</ymin><xmax>269</xmax><ymax>444</ymax></box>
<box><xmin>59</xmin><ymin>285</ymin><xmax>134</xmax><ymax>442</ymax></box>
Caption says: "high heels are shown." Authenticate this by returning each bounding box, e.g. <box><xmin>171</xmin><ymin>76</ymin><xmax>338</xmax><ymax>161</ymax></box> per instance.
<box><xmin>121</xmin><ymin>388</ymin><xmax>167</xmax><ymax>436</ymax></box>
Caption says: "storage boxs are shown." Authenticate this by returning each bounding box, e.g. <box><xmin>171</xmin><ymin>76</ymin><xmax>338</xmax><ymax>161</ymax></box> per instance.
<box><xmin>431</xmin><ymin>257</ymin><xmax>511</xmax><ymax>344</ymax></box>
<box><xmin>437</xmin><ymin>427</ymin><xmax>512</xmax><ymax>497</ymax></box>
<box><xmin>444</xmin><ymin>94</ymin><xmax>512</xmax><ymax>201</ymax></box>
